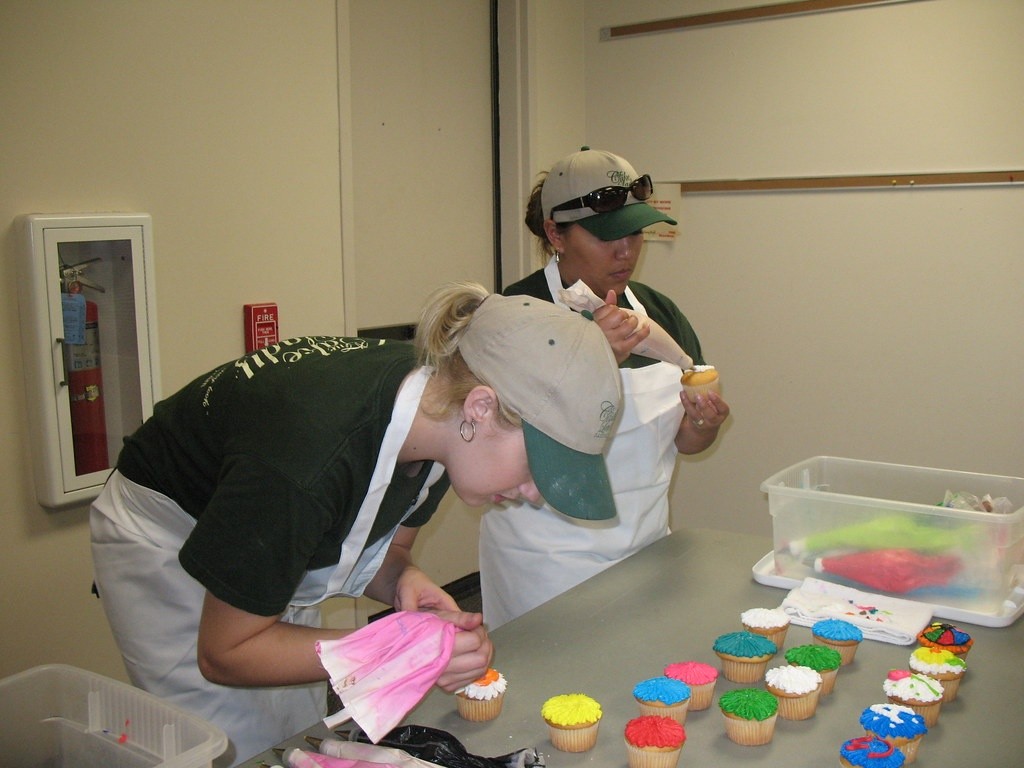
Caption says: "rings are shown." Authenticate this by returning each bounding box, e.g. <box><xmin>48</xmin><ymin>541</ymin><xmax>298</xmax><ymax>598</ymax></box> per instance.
<box><xmin>697</xmin><ymin>420</ymin><xmax>704</xmax><ymax>425</ymax></box>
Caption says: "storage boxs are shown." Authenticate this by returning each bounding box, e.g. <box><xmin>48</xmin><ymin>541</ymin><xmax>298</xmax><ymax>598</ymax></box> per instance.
<box><xmin>0</xmin><ymin>663</ymin><xmax>230</xmax><ymax>768</ymax></box>
<box><xmin>749</xmin><ymin>453</ymin><xmax>1024</xmax><ymax>629</ymax></box>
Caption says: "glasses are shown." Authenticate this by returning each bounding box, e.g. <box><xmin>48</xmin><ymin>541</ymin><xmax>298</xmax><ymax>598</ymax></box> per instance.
<box><xmin>549</xmin><ymin>173</ymin><xmax>655</xmax><ymax>221</ymax></box>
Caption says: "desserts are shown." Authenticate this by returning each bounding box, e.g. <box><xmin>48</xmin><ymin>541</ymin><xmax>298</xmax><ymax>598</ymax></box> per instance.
<box><xmin>681</xmin><ymin>365</ymin><xmax>718</xmax><ymax>403</ymax></box>
<box><xmin>542</xmin><ymin>694</ymin><xmax>602</xmax><ymax>752</ymax></box>
<box><xmin>455</xmin><ymin>669</ymin><xmax>507</xmax><ymax>723</ymax></box>
<box><xmin>624</xmin><ymin>607</ymin><xmax>863</xmax><ymax>768</ymax></box>
<box><xmin>839</xmin><ymin>622</ymin><xmax>973</xmax><ymax>768</ymax></box>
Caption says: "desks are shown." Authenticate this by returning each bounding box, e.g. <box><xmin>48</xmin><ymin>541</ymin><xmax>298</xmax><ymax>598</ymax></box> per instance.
<box><xmin>226</xmin><ymin>528</ymin><xmax>1024</xmax><ymax>768</ymax></box>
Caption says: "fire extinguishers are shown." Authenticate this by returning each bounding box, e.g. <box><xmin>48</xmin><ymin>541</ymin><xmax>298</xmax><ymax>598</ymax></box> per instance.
<box><xmin>59</xmin><ymin>258</ymin><xmax>109</xmax><ymax>477</ymax></box>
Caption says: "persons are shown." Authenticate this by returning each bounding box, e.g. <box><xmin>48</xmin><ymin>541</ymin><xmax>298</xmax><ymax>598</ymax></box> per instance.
<box><xmin>472</xmin><ymin>143</ymin><xmax>730</xmax><ymax>627</ymax></box>
<box><xmin>89</xmin><ymin>282</ymin><xmax>628</xmax><ymax>766</ymax></box>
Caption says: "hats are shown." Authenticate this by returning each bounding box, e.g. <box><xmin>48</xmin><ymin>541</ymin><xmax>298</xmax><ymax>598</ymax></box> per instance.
<box><xmin>458</xmin><ymin>294</ymin><xmax>626</xmax><ymax>529</ymax></box>
<box><xmin>541</xmin><ymin>146</ymin><xmax>678</xmax><ymax>242</ymax></box>
